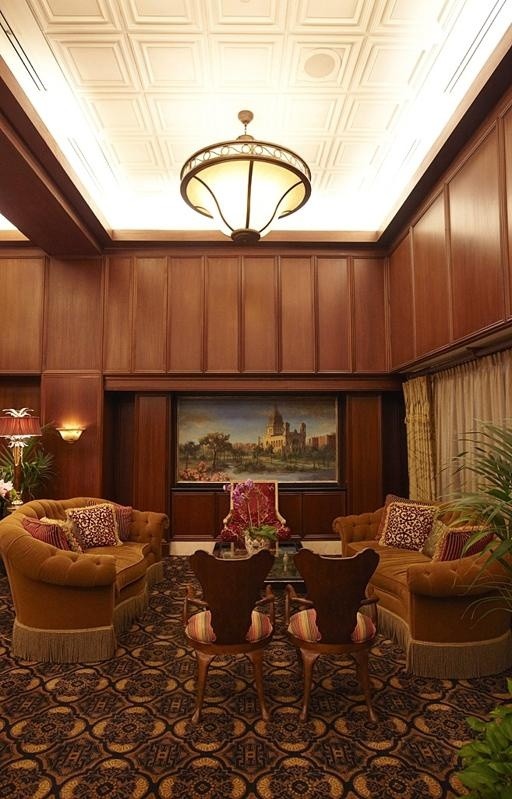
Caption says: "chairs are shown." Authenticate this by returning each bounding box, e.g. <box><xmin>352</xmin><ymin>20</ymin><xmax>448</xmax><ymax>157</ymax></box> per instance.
<box><xmin>219</xmin><ymin>482</ymin><xmax>290</xmax><ymax>548</ymax></box>
<box><xmin>183</xmin><ymin>550</ymin><xmax>276</xmax><ymax>724</ymax></box>
<box><xmin>285</xmin><ymin>547</ymin><xmax>380</xmax><ymax>723</ymax></box>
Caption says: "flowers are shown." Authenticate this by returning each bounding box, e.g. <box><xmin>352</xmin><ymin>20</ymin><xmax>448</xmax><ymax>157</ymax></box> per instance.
<box><xmin>223</xmin><ymin>479</ymin><xmax>280</xmax><ymax>541</ymax></box>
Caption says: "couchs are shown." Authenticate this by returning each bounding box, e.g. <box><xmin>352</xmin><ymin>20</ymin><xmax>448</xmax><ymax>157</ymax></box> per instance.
<box><xmin>332</xmin><ymin>494</ymin><xmax>512</xmax><ymax>680</ymax></box>
<box><xmin>0</xmin><ymin>497</ymin><xmax>171</xmax><ymax>664</ymax></box>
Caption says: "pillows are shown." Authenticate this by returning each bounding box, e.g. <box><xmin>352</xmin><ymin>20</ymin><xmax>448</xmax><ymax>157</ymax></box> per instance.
<box><xmin>379</xmin><ymin>501</ymin><xmax>441</xmax><ymax>549</ymax></box>
<box><xmin>64</xmin><ymin>505</ymin><xmax>119</xmax><ymax>547</ymax></box>
<box><xmin>115</xmin><ymin>504</ymin><xmax>132</xmax><ymax>542</ymax></box>
<box><xmin>374</xmin><ymin>494</ymin><xmax>433</xmax><ymax>541</ymax></box>
<box><xmin>422</xmin><ymin>519</ymin><xmax>449</xmax><ymax>558</ymax></box>
<box><xmin>430</xmin><ymin>524</ymin><xmax>492</xmax><ymax>561</ymax></box>
<box><xmin>24</xmin><ymin>516</ymin><xmax>82</xmax><ymax>554</ymax></box>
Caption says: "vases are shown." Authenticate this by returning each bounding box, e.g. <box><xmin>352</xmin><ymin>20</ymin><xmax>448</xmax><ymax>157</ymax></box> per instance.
<box><xmin>245</xmin><ymin>538</ymin><xmax>276</xmax><ymax>558</ymax></box>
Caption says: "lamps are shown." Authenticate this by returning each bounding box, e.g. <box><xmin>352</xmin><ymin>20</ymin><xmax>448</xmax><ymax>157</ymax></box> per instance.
<box><xmin>0</xmin><ymin>405</ymin><xmax>42</xmax><ymax>510</ymax></box>
<box><xmin>179</xmin><ymin>110</ymin><xmax>310</xmax><ymax>242</ymax></box>
<box><xmin>55</xmin><ymin>428</ymin><xmax>85</xmax><ymax>445</ymax></box>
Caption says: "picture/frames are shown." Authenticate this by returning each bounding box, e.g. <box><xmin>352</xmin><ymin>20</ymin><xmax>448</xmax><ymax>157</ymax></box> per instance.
<box><xmin>171</xmin><ymin>392</ymin><xmax>344</xmax><ymax>490</ymax></box>
